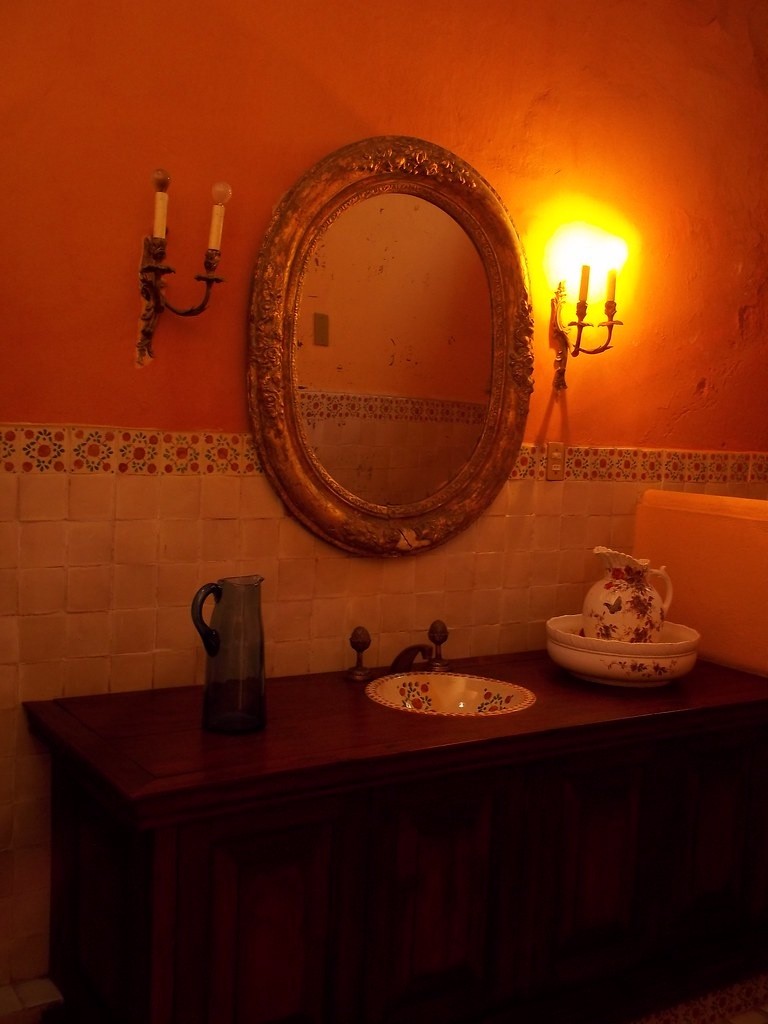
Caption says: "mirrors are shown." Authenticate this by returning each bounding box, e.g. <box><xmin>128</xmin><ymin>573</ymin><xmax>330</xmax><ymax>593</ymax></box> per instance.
<box><xmin>243</xmin><ymin>134</ymin><xmax>537</xmax><ymax>560</ymax></box>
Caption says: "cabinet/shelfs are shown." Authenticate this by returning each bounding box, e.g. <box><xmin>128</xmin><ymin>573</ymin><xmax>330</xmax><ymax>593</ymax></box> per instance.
<box><xmin>48</xmin><ymin>723</ymin><xmax>767</xmax><ymax>1024</ymax></box>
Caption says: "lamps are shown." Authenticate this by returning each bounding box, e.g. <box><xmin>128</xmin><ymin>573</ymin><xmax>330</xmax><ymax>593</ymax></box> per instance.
<box><xmin>136</xmin><ymin>168</ymin><xmax>232</xmax><ymax>367</ymax></box>
<box><xmin>550</xmin><ymin>266</ymin><xmax>623</xmax><ymax>394</ymax></box>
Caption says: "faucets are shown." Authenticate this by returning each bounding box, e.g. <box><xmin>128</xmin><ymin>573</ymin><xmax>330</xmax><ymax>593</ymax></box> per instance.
<box><xmin>389</xmin><ymin>644</ymin><xmax>433</xmax><ymax>672</ymax></box>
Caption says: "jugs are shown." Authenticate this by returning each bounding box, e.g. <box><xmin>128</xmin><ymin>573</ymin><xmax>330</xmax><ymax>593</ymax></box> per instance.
<box><xmin>191</xmin><ymin>575</ymin><xmax>265</xmax><ymax>735</ymax></box>
<box><xmin>583</xmin><ymin>546</ymin><xmax>673</xmax><ymax>643</ymax></box>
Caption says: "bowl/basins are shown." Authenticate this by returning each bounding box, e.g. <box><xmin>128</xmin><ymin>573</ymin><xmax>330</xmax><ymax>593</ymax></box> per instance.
<box><xmin>545</xmin><ymin>613</ymin><xmax>700</xmax><ymax>687</ymax></box>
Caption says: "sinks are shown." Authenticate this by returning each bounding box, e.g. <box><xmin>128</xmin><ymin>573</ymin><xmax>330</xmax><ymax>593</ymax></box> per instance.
<box><xmin>364</xmin><ymin>671</ymin><xmax>538</xmax><ymax>717</ymax></box>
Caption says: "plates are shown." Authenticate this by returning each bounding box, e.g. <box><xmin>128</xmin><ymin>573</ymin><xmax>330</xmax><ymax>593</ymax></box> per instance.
<box><xmin>365</xmin><ymin>671</ymin><xmax>536</xmax><ymax>720</ymax></box>
